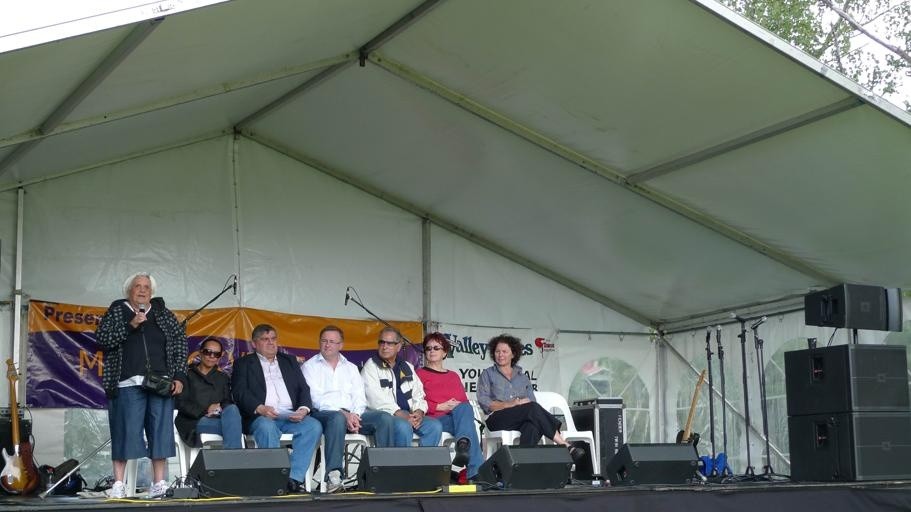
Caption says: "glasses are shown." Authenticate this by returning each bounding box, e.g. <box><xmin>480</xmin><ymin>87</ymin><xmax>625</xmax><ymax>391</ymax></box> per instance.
<box><xmin>203</xmin><ymin>349</ymin><xmax>221</xmax><ymax>358</ymax></box>
<box><xmin>321</xmin><ymin>339</ymin><xmax>341</xmax><ymax>344</ymax></box>
<box><xmin>378</xmin><ymin>340</ymin><xmax>400</xmax><ymax>345</ymax></box>
<box><xmin>425</xmin><ymin>345</ymin><xmax>443</xmax><ymax>351</ymax></box>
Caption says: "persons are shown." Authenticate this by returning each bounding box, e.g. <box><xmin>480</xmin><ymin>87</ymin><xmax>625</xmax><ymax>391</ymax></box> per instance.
<box><xmin>94</xmin><ymin>272</ymin><xmax>188</xmax><ymax>498</ymax></box>
<box><xmin>231</xmin><ymin>325</ymin><xmax>323</xmax><ymax>495</ymax></box>
<box><xmin>300</xmin><ymin>325</ymin><xmax>394</xmax><ymax>493</ymax></box>
<box><xmin>476</xmin><ymin>333</ymin><xmax>585</xmax><ymax>461</ymax></box>
<box><xmin>414</xmin><ymin>331</ymin><xmax>485</xmax><ymax>485</ymax></box>
<box><xmin>362</xmin><ymin>326</ymin><xmax>443</xmax><ymax>447</ymax></box>
<box><xmin>172</xmin><ymin>336</ymin><xmax>243</xmax><ymax>449</ymax></box>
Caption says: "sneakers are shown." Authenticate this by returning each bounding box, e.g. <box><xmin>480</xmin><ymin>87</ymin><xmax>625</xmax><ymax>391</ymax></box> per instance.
<box><xmin>110</xmin><ymin>481</ymin><xmax>127</xmax><ymax>498</ymax></box>
<box><xmin>150</xmin><ymin>480</ymin><xmax>169</xmax><ymax>497</ymax></box>
<box><xmin>326</xmin><ymin>469</ymin><xmax>344</xmax><ymax>494</ymax></box>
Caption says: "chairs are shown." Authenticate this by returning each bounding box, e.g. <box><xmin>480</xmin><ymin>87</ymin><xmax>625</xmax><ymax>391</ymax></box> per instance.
<box><xmin>482</xmin><ymin>412</ymin><xmax>548</xmax><ymax>457</ymax></box>
<box><xmin>320</xmin><ymin>431</ymin><xmax>369</xmax><ymax>492</ymax></box>
<box><xmin>534</xmin><ymin>388</ymin><xmax>599</xmax><ymax>484</ymax></box>
<box><xmin>248</xmin><ymin>431</ymin><xmax>318</xmax><ymax>493</ymax></box>
<box><xmin>173</xmin><ymin>409</ymin><xmax>245</xmax><ymax>485</ymax></box>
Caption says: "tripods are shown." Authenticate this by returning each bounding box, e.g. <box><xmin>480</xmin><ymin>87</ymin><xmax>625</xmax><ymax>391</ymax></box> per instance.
<box><xmin>710</xmin><ymin>343</ymin><xmax>758</xmax><ymax>484</ymax></box>
<box><xmin>723</xmin><ymin>321</ymin><xmax>769</xmax><ymax>482</ymax></box>
<box><xmin>695</xmin><ymin>342</ymin><xmax>739</xmax><ymax>484</ymax></box>
<box><xmin>741</xmin><ymin>332</ymin><xmax>790</xmax><ymax>483</ymax></box>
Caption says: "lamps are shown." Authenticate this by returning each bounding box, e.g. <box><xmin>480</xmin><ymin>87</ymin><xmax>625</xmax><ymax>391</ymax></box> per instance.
<box><xmin>481</xmin><ymin>440</ymin><xmax>576</xmax><ymax>489</ymax></box>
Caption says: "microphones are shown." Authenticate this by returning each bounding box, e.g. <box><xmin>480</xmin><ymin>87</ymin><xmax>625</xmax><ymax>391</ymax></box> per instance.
<box><xmin>716</xmin><ymin>324</ymin><xmax>721</xmax><ymax>343</ymax></box>
<box><xmin>233</xmin><ymin>276</ymin><xmax>238</xmax><ymax>295</ymax></box>
<box><xmin>706</xmin><ymin>326</ymin><xmax>711</xmax><ymax>343</ymax></box>
<box><xmin>751</xmin><ymin>316</ymin><xmax>767</xmax><ymax>329</ymax></box>
<box><xmin>729</xmin><ymin>312</ymin><xmax>745</xmax><ymax>323</ymax></box>
<box><xmin>139</xmin><ymin>303</ymin><xmax>146</xmax><ymax>333</ymax></box>
<box><xmin>344</xmin><ymin>287</ymin><xmax>350</xmax><ymax>305</ymax></box>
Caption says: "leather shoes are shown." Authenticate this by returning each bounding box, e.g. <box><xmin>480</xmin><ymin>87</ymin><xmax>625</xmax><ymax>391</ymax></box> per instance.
<box><xmin>568</xmin><ymin>445</ymin><xmax>586</xmax><ymax>458</ymax></box>
<box><xmin>452</xmin><ymin>442</ymin><xmax>470</xmax><ymax>467</ymax></box>
<box><xmin>289</xmin><ymin>479</ymin><xmax>306</xmax><ymax>493</ymax></box>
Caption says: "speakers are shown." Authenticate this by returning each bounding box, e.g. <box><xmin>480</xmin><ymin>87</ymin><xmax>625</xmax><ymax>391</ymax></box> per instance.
<box><xmin>783</xmin><ymin>344</ymin><xmax>910</xmax><ymax>482</ymax></box>
<box><xmin>476</xmin><ymin>443</ymin><xmax>573</xmax><ymax>492</ymax></box>
<box><xmin>805</xmin><ymin>282</ymin><xmax>902</xmax><ymax>333</ymax></box>
